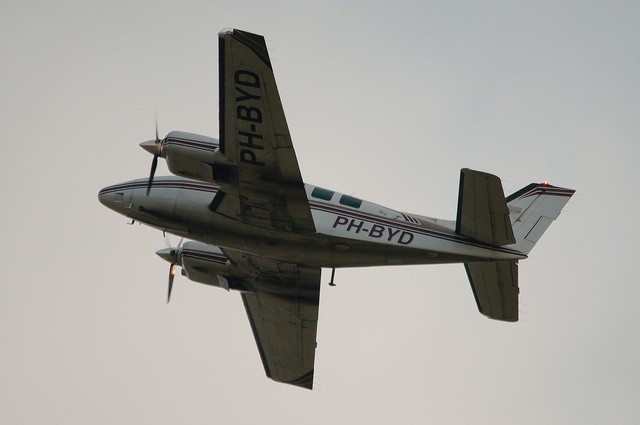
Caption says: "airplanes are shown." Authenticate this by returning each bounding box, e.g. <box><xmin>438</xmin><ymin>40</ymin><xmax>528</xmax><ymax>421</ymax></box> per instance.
<box><xmin>96</xmin><ymin>27</ymin><xmax>577</xmax><ymax>390</ymax></box>
